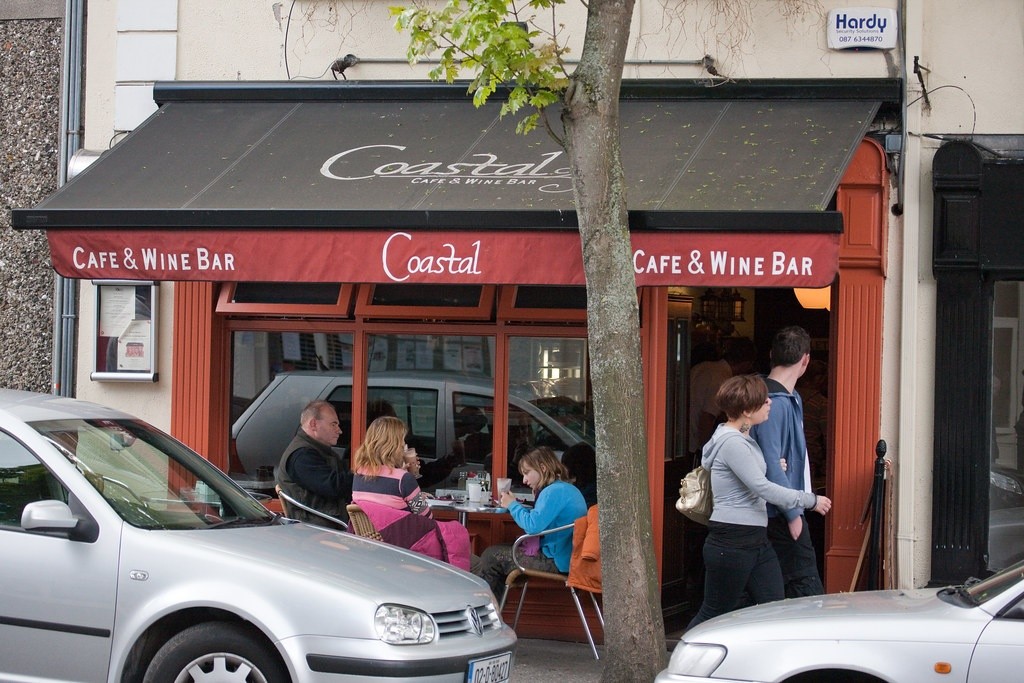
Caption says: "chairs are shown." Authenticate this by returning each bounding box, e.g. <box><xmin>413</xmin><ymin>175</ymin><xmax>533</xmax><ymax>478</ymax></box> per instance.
<box><xmin>346</xmin><ymin>499</ymin><xmax>471</xmax><ymax>573</ymax></box>
<box><xmin>275</xmin><ymin>485</ymin><xmax>349</xmax><ymax>530</ymax></box>
<box><xmin>461</xmin><ymin>407</ymin><xmax>492</xmax><ymax>463</ymax></box>
<box><xmin>497</xmin><ymin>504</ymin><xmax>605</xmax><ymax>661</ymax></box>
<box><xmin>0</xmin><ymin>468</ymin><xmax>37</xmax><ymax>521</ymax></box>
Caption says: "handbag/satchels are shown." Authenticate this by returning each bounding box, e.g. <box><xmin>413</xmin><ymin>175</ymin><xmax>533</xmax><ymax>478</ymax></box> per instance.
<box><xmin>674</xmin><ymin>466</ymin><xmax>712</xmax><ymax>525</ymax></box>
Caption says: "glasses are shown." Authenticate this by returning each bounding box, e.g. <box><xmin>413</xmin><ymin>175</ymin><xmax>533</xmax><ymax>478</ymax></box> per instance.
<box><xmin>762</xmin><ymin>397</ymin><xmax>768</xmax><ymax>405</ymax></box>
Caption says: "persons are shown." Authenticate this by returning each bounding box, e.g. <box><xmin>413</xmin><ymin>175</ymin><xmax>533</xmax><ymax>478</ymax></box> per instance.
<box><xmin>478</xmin><ymin>446</ymin><xmax>587</xmax><ymax>608</ymax></box>
<box><xmin>278</xmin><ymin>401</ymin><xmax>352</xmax><ymax>531</ymax></box>
<box><xmin>352</xmin><ymin>417</ymin><xmax>434</xmax><ymax>520</ymax></box>
<box><xmin>686</xmin><ymin>313</ymin><xmax>832</xmax><ymax>632</ymax></box>
<box><xmin>342</xmin><ymin>400</ymin><xmax>459</xmax><ymax>488</ymax></box>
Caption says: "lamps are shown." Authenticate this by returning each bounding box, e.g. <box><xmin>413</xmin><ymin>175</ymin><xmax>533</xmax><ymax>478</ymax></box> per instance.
<box><xmin>692</xmin><ymin>288</ymin><xmax>748</xmax><ymax>322</ymax></box>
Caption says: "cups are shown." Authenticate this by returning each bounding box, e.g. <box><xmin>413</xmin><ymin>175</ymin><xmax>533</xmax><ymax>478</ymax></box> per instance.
<box><xmin>403</xmin><ymin>448</ymin><xmax>422</xmax><ymax>478</ymax></box>
<box><xmin>469</xmin><ymin>485</ymin><xmax>482</xmax><ymax>502</ymax></box>
<box><xmin>497</xmin><ymin>478</ymin><xmax>512</xmax><ymax>507</ymax></box>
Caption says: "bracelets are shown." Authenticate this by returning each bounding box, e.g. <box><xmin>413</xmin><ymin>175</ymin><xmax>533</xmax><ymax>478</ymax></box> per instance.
<box><xmin>805</xmin><ymin>492</ymin><xmax>817</xmax><ymax>510</ymax></box>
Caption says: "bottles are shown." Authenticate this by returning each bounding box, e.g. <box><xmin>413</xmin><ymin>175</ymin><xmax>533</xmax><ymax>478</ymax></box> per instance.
<box><xmin>458</xmin><ymin>472</ymin><xmax>468</xmax><ymax>491</ymax></box>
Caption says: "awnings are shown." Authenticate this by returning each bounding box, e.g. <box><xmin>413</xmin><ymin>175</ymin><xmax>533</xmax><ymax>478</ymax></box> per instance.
<box><xmin>12</xmin><ymin>78</ymin><xmax>903</xmax><ymax>289</ymax></box>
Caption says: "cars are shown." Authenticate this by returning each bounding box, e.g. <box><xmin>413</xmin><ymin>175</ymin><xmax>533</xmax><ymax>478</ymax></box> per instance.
<box><xmin>656</xmin><ymin>558</ymin><xmax>1024</xmax><ymax>683</ymax></box>
<box><xmin>987</xmin><ymin>507</ymin><xmax>1024</xmax><ymax>570</ymax></box>
<box><xmin>991</xmin><ymin>464</ymin><xmax>1024</xmax><ymax>506</ymax></box>
<box><xmin>0</xmin><ymin>388</ymin><xmax>519</xmax><ymax>683</ymax></box>
<box><xmin>233</xmin><ymin>371</ymin><xmax>596</xmax><ymax>493</ymax></box>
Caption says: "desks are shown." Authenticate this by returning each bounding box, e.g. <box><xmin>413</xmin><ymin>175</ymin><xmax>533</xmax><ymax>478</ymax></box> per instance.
<box><xmin>139</xmin><ymin>490</ymin><xmax>272</xmax><ymax>514</ymax></box>
<box><xmin>424</xmin><ymin>499</ymin><xmax>532</xmax><ymax>529</ymax></box>
